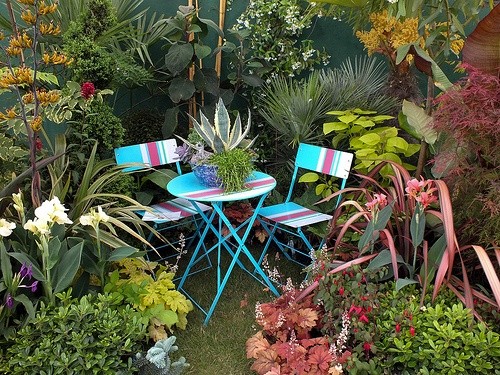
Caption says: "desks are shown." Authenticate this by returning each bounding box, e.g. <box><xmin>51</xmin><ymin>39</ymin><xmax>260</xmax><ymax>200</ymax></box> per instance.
<box><xmin>166</xmin><ymin>171</ymin><xmax>281</xmax><ymax>327</ymax></box>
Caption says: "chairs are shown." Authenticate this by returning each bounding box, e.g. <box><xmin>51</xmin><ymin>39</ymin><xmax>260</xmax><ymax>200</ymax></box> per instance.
<box><xmin>114</xmin><ymin>139</ymin><xmax>213</xmax><ymax>283</ymax></box>
<box><xmin>252</xmin><ymin>143</ymin><xmax>353</xmax><ymax>286</ymax></box>
